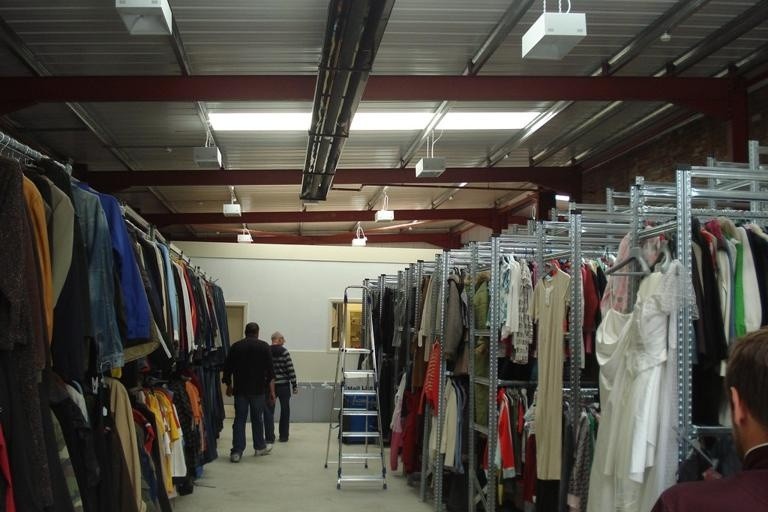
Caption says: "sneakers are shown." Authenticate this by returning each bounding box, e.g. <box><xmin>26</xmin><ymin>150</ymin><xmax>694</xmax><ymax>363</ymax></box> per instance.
<box><xmin>230</xmin><ymin>454</ymin><xmax>241</xmax><ymax>463</ymax></box>
<box><xmin>265</xmin><ymin>439</ymin><xmax>289</xmax><ymax>443</ymax></box>
<box><xmin>255</xmin><ymin>444</ymin><xmax>273</xmax><ymax>457</ymax></box>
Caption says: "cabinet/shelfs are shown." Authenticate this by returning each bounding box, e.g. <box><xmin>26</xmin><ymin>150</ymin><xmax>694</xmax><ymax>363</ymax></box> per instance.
<box><xmin>363</xmin><ymin>141</ymin><xmax>768</xmax><ymax>511</ymax></box>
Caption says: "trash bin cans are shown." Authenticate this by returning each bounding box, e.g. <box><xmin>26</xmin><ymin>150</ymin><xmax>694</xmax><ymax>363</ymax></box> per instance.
<box><xmin>342</xmin><ymin>389</ymin><xmax>376</xmax><ymax>445</ymax></box>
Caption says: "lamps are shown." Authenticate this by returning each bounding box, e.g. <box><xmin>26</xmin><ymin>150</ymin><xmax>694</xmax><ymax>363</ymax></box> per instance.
<box><xmin>352</xmin><ymin>192</ymin><xmax>394</xmax><ymax>246</ymax></box>
<box><xmin>414</xmin><ymin>135</ymin><xmax>446</xmax><ymax>180</ymax></box>
<box><xmin>223</xmin><ymin>194</ymin><xmax>252</xmax><ymax>244</ymax></box>
<box><xmin>194</xmin><ymin>130</ymin><xmax>222</xmax><ymax>170</ymax></box>
<box><xmin>521</xmin><ymin>1</ymin><xmax>587</xmax><ymax>61</ymax></box>
<box><xmin>115</xmin><ymin>1</ymin><xmax>174</xmax><ymax>37</ymax></box>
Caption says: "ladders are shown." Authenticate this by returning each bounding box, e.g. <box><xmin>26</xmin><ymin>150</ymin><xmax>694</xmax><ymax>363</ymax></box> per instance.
<box><xmin>324</xmin><ymin>284</ymin><xmax>388</xmax><ymax>490</ymax></box>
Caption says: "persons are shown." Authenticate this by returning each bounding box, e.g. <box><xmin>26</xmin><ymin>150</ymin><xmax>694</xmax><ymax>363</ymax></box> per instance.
<box><xmin>647</xmin><ymin>324</ymin><xmax>767</xmax><ymax>512</ymax></box>
<box><xmin>221</xmin><ymin>323</ymin><xmax>278</xmax><ymax>463</ymax></box>
<box><xmin>263</xmin><ymin>331</ymin><xmax>299</xmax><ymax>444</ymax></box>
